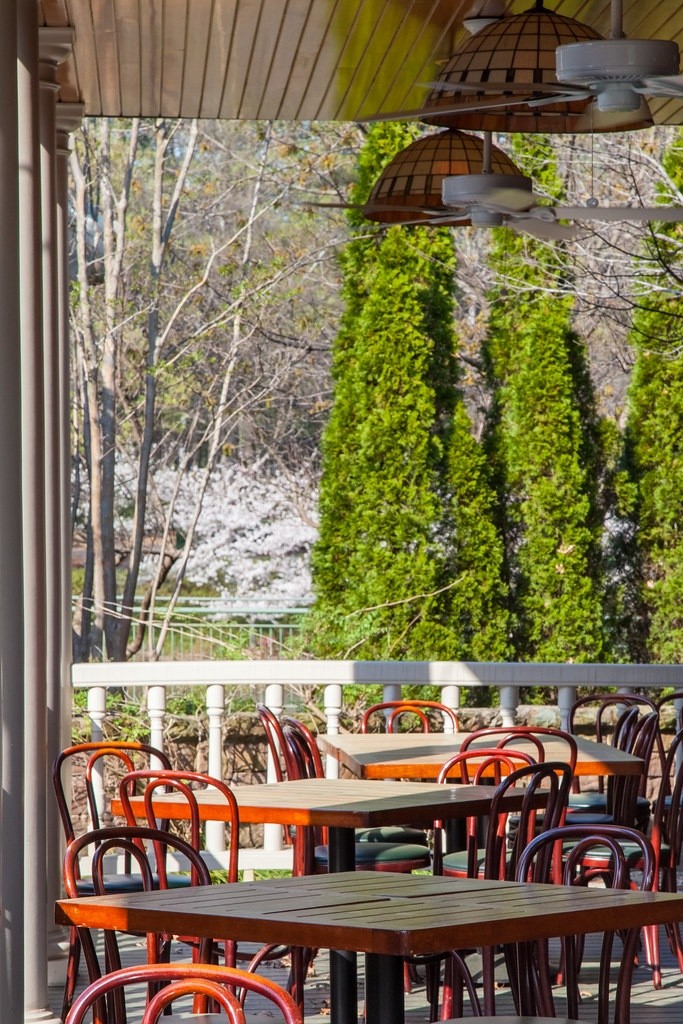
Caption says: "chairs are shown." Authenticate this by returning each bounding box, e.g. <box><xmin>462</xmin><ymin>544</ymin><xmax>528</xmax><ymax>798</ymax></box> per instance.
<box><xmin>55</xmin><ymin>692</ymin><xmax>683</xmax><ymax>1022</ymax></box>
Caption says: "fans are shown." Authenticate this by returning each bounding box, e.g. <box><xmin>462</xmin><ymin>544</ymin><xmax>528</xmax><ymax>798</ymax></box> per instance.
<box><xmin>349</xmin><ymin>0</ymin><xmax>683</xmax><ymax>242</ymax></box>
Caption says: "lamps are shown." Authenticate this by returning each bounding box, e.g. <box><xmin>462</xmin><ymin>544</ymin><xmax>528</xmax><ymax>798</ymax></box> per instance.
<box><xmin>421</xmin><ymin>1</ymin><xmax>659</xmax><ymax>133</ymax></box>
<box><xmin>365</xmin><ymin>130</ymin><xmax>523</xmax><ymax>227</ymax></box>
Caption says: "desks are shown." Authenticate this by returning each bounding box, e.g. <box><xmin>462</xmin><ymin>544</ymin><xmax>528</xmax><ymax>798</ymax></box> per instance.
<box><xmin>310</xmin><ymin>729</ymin><xmax>644</xmax><ymax>856</ymax></box>
<box><xmin>53</xmin><ymin>869</ymin><xmax>681</xmax><ymax>1024</ymax></box>
<box><xmin>108</xmin><ymin>778</ymin><xmax>562</xmax><ymax>1023</ymax></box>
